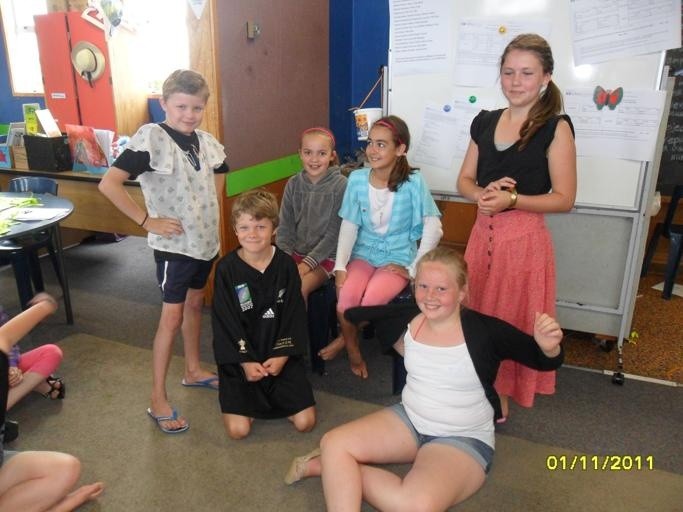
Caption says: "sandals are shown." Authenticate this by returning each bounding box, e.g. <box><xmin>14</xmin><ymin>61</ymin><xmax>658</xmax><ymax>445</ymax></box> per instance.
<box><xmin>40</xmin><ymin>374</ymin><xmax>65</xmax><ymax>400</ymax></box>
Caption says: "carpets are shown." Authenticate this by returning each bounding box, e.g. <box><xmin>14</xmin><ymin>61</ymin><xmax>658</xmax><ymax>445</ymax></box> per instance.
<box><xmin>1</xmin><ymin>332</ymin><xmax>682</xmax><ymax>511</ymax></box>
<box><xmin>560</xmin><ymin>260</ymin><xmax>683</xmax><ymax>390</ymax></box>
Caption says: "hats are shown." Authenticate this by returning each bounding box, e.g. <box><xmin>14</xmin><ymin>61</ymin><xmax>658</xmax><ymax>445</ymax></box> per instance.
<box><xmin>70</xmin><ymin>40</ymin><xmax>106</xmax><ymax>83</ymax></box>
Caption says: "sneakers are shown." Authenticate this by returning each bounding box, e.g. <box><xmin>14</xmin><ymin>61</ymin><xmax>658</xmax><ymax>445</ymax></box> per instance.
<box><xmin>285</xmin><ymin>447</ymin><xmax>320</xmax><ymax>485</ymax></box>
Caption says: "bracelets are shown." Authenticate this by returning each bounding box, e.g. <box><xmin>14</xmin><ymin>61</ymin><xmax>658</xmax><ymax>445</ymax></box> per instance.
<box><xmin>507</xmin><ymin>188</ymin><xmax>517</xmax><ymax>209</ymax></box>
<box><xmin>36</xmin><ymin>299</ymin><xmax>57</xmax><ymax>311</ymax></box>
<box><xmin>139</xmin><ymin>213</ymin><xmax>148</xmax><ymax>227</ymax></box>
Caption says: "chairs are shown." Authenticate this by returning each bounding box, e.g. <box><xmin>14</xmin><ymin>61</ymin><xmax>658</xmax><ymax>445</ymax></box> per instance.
<box><xmin>0</xmin><ymin>176</ymin><xmax>68</xmax><ymax>297</ymax></box>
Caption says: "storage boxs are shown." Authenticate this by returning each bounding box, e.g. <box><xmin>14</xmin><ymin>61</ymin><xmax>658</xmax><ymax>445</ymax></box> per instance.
<box><xmin>11</xmin><ymin>144</ymin><xmax>30</xmax><ymax>169</ymax></box>
<box><xmin>0</xmin><ymin>134</ymin><xmax>12</xmax><ymax>169</ymax></box>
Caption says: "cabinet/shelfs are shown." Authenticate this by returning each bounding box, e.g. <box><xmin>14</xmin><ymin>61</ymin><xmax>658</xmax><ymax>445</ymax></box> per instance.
<box><xmin>32</xmin><ymin>10</ymin><xmax>150</xmax><ymax>143</ymax></box>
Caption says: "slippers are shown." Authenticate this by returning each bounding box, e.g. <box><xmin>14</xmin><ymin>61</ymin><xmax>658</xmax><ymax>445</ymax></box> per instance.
<box><xmin>146</xmin><ymin>407</ymin><xmax>189</xmax><ymax>433</ymax></box>
<box><xmin>182</xmin><ymin>371</ymin><xmax>219</xmax><ymax>390</ymax></box>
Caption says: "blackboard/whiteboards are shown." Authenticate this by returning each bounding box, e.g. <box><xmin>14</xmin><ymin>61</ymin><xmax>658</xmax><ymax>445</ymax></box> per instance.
<box><xmin>381</xmin><ymin>47</ymin><xmax>668</xmax><ymax>213</ymax></box>
<box><xmin>654</xmin><ymin>46</ymin><xmax>683</xmax><ymax>192</ymax></box>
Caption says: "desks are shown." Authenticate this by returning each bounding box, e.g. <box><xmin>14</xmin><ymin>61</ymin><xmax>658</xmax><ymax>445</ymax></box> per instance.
<box><xmin>0</xmin><ymin>164</ymin><xmax>148</xmax><ymax>259</ymax></box>
<box><xmin>0</xmin><ymin>191</ymin><xmax>77</xmax><ymax>327</ymax></box>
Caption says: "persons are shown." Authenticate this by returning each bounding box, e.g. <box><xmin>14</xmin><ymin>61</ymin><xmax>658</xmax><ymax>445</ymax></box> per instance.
<box><xmin>98</xmin><ymin>70</ymin><xmax>229</xmax><ymax>435</ymax></box>
<box><xmin>275</xmin><ymin>128</ymin><xmax>349</xmax><ymax>313</ymax></box>
<box><xmin>284</xmin><ymin>248</ymin><xmax>564</xmax><ymax>512</ymax></box>
<box><xmin>213</xmin><ymin>191</ymin><xmax>316</xmax><ymax>440</ymax></box>
<box><xmin>317</xmin><ymin>116</ymin><xmax>444</xmax><ymax>378</ymax></box>
<box><xmin>458</xmin><ymin>35</ymin><xmax>576</xmax><ymax>424</ymax></box>
<box><xmin>0</xmin><ymin>290</ymin><xmax>104</xmax><ymax>512</ymax></box>
<box><xmin>0</xmin><ymin>304</ymin><xmax>65</xmax><ymax>443</ymax></box>
